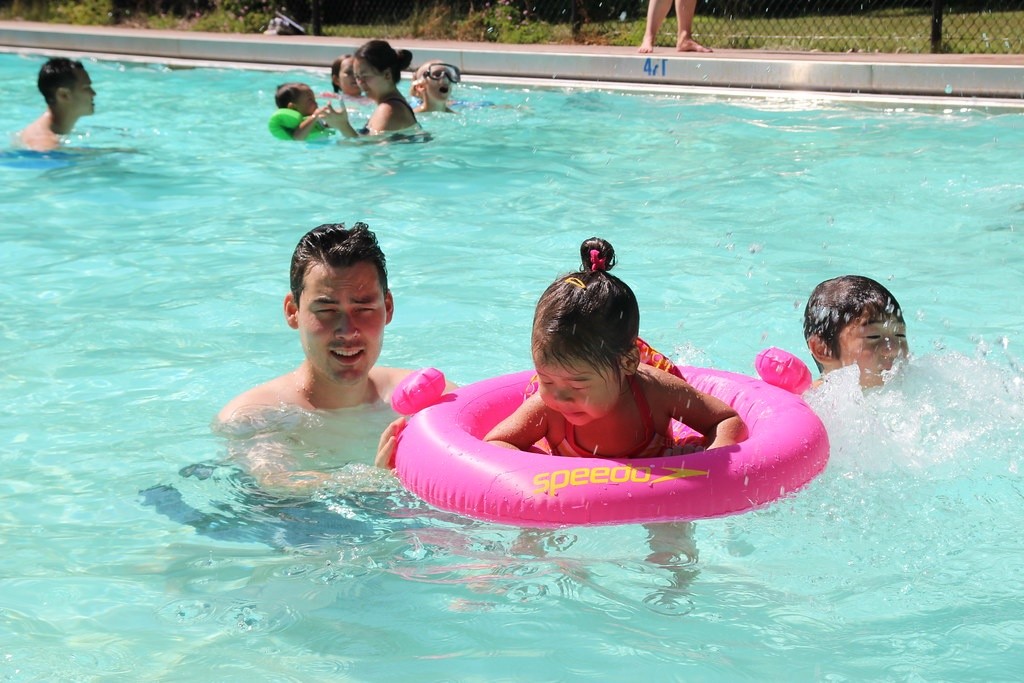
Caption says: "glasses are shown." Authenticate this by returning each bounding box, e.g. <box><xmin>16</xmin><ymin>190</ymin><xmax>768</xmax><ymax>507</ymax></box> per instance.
<box><xmin>422</xmin><ymin>62</ymin><xmax>462</xmax><ymax>84</ymax></box>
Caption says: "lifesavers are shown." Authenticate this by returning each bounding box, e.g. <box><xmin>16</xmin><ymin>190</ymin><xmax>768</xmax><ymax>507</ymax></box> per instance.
<box><xmin>267</xmin><ymin>107</ymin><xmax>336</xmax><ymax>139</ymax></box>
<box><xmin>390</xmin><ymin>344</ymin><xmax>831</xmax><ymax>528</ymax></box>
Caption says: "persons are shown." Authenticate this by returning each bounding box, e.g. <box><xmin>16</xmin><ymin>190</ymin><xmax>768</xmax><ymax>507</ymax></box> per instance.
<box><xmin>275</xmin><ymin>83</ymin><xmax>330</xmax><ymax>140</ymax></box>
<box><xmin>803</xmin><ymin>273</ymin><xmax>910</xmax><ymax>392</ymax></box>
<box><xmin>318</xmin><ymin>39</ymin><xmax>417</xmax><ymax>136</ymax></box>
<box><xmin>25</xmin><ymin>56</ymin><xmax>96</xmax><ymax>150</ymax></box>
<box><xmin>483</xmin><ymin>236</ymin><xmax>743</xmax><ymax>457</ymax></box>
<box><xmin>639</xmin><ymin>0</ymin><xmax>713</xmax><ymax>52</ymax></box>
<box><xmin>331</xmin><ymin>55</ymin><xmax>362</xmax><ymax>96</ymax></box>
<box><xmin>408</xmin><ymin>59</ymin><xmax>457</xmax><ymax>113</ymax></box>
<box><xmin>218</xmin><ymin>223</ymin><xmax>462</xmax><ymax>468</ymax></box>
